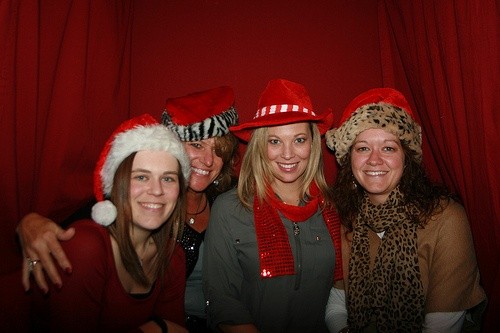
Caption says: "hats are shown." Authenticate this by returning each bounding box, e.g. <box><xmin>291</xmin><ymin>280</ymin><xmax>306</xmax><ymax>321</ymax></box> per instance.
<box><xmin>325</xmin><ymin>87</ymin><xmax>422</xmax><ymax>168</ymax></box>
<box><xmin>161</xmin><ymin>88</ymin><xmax>240</xmax><ymax>142</ymax></box>
<box><xmin>229</xmin><ymin>79</ymin><xmax>334</xmax><ymax>142</ymax></box>
<box><xmin>91</xmin><ymin>114</ymin><xmax>192</xmax><ymax>226</ymax></box>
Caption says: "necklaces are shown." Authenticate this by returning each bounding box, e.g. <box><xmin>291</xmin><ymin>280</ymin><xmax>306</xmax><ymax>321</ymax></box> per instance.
<box><xmin>187</xmin><ymin>199</ymin><xmax>207</xmax><ymax>216</ymax></box>
<box><xmin>187</xmin><ymin>192</ymin><xmax>203</xmax><ymax>225</ymax></box>
<box><xmin>140</xmin><ymin>238</ymin><xmax>150</xmax><ymax>266</ymax></box>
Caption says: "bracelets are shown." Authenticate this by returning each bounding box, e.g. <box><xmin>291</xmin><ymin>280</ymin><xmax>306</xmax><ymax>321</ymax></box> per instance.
<box><xmin>156</xmin><ymin>318</ymin><xmax>168</xmax><ymax>333</ymax></box>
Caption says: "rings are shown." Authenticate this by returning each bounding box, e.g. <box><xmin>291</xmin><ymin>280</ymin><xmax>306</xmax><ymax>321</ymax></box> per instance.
<box><xmin>27</xmin><ymin>257</ymin><xmax>40</xmax><ymax>269</ymax></box>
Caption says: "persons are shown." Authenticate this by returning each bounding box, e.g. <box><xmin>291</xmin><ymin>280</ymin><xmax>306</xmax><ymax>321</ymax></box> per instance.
<box><xmin>16</xmin><ymin>86</ymin><xmax>238</xmax><ymax>333</ymax></box>
<box><xmin>201</xmin><ymin>78</ymin><xmax>343</xmax><ymax>333</ymax></box>
<box><xmin>326</xmin><ymin>88</ymin><xmax>487</xmax><ymax>333</ymax></box>
<box><xmin>32</xmin><ymin>113</ymin><xmax>191</xmax><ymax>333</ymax></box>
